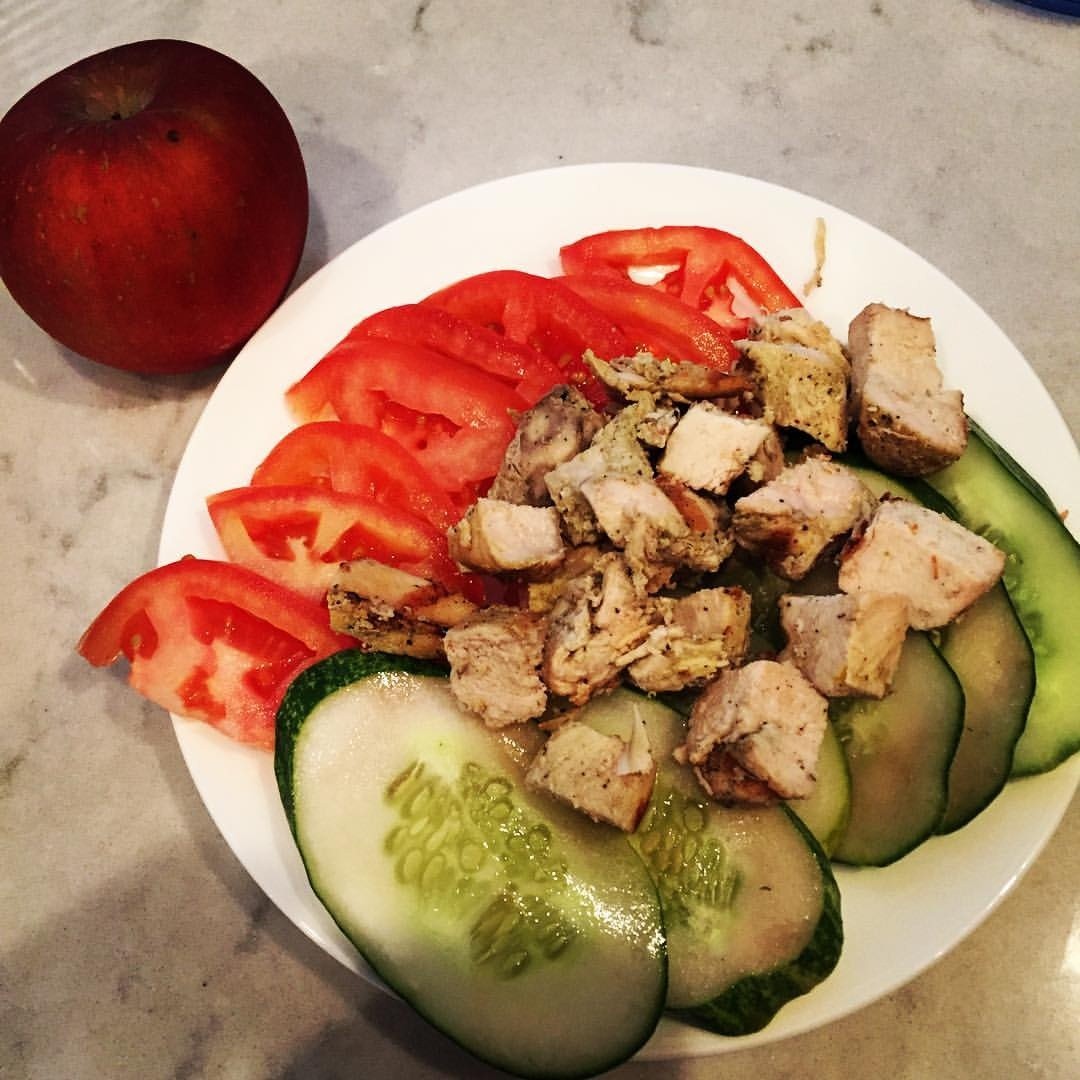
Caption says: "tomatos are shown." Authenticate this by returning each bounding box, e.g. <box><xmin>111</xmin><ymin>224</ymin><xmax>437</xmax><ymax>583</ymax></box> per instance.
<box><xmin>74</xmin><ymin>224</ymin><xmax>820</xmax><ymax>758</ymax></box>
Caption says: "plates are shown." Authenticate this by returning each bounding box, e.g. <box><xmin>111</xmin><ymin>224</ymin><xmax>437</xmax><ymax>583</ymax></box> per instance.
<box><xmin>159</xmin><ymin>163</ymin><xmax>1080</xmax><ymax>1061</ymax></box>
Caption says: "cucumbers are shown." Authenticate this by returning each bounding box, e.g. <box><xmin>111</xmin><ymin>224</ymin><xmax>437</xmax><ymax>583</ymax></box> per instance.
<box><xmin>271</xmin><ymin>418</ymin><xmax>1080</xmax><ymax>1080</ymax></box>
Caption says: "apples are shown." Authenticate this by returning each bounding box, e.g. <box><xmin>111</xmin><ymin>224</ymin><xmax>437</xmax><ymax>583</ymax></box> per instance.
<box><xmin>0</xmin><ymin>38</ymin><xmax>308</xmax><ymax>377</ymax></box>
